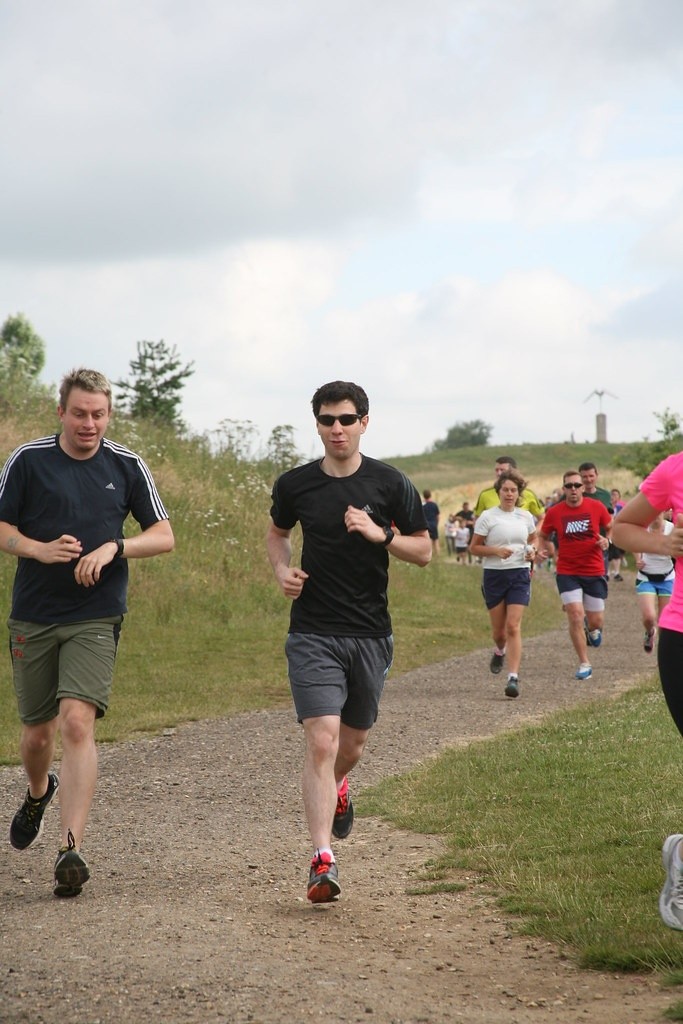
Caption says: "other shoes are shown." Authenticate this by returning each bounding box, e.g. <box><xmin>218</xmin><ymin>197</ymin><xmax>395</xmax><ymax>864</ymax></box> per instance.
<box><xmin>457</xmin><ymin>556</ymin><xmax>460</xmax><ymax>562</ymax></box>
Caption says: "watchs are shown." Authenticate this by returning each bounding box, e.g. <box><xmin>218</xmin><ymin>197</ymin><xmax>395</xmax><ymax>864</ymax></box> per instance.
<box><xmin>380</xmin><ymin>526</ymin><xmax>394</xmax><ymax>547</ymax></box>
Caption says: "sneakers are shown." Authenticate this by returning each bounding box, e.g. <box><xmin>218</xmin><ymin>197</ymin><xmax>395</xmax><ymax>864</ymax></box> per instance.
<box><xmin>306</xmin><ymin>847</ymin><xmax>341</xmax><ymax>904</ymax></box>
<box><xmin>52</xmin><ymin>847</ymin><xmax>91</xmax><ymax>898</ymax></box>
<box><xmin>504</xmin><ymin>676</ymin><xmax>519</xmax><ymax>698</ymax></box>
<box><xmin>8</xmin><ymin>772</ymin><xmax>61</xmax><ymax>852</ymax></box>
<box><xmin>643</xmin><ymin>626</ymin><xmax>656</xmax><ymax>653</ymax></box>
<box><xmin>584</xmin><ymin>615</ymin><xmax>602</xmax><ymax>648</ymax></box>
<box><xmin>658</xmin><ymin>832</ymin><xmax>683</xmax><ymax>932</ymax></box>
<box><xmin>613</xmin><ymin>574</ymin><xmax>623</xmax><ymax>582</ymax></box>
<box><xmin>604</xmin><ymin>575</ymin><xmax>609</xmax><ymax>582</ymax></box>
<box><xmin>331</xmin><ymin>775</ymin><xmax>354</xmax><ymax>840</ymax></box>
<box><xmin>489</xmin><ymin>648</ymin><xmax>506</xmax><ymax>674</ymax></box>
<box><xmin>574</xmin><ymin>666</ymin><xmax>592</xmax><ymax>681</ymax></box>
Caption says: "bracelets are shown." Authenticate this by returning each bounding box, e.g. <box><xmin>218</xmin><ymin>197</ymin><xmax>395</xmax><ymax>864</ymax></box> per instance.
<box><xmin>607</xmin><ymin>537</ymin><xmax>613</xmax><ymax>547</ymax></box>
<box><xmin>110</xmin><ymin>538</ymin><xmax>123</xmax><ymax>560</ymax></box>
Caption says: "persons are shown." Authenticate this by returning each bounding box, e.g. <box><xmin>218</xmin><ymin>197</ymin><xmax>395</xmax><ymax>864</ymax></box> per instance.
<box><xmin>469</xmin><ymin>471</ymin><xmax>539</xmax><ymax>698</ymax></box>
<box><xmin>538</xmin><ymin>472</ymin><xmax>614</xmax><ymax>680</ymax></box>
<box><xmin>473</xmin><ymin>457</ymin><xmax>546</xmax><ymax>575</ymax></box>
<box><xmin>268</xmin><ymin>382</ymin><xmax>433</xmax><ymax>904</ymax></box>
<box><xmin>421</xmin><ymin>487</ymin><xmax>641</xmax><ymax>582</ymax></box>
<box><xmin>631</xmin><ymin>504</ymin><xmax>676</xmax><ymax>653</ymax></box>
<box><xmin>0</xmin><ymin>369</ymin><xmax>175</xmax><ymax>899</ymax></box>
<box><xmin>611</xmin><ymin>451</ymin><xmax>683</xmax><ymax>930</ymax></box>
<box><xmin>557</xmin><ymin>462</ymin><xmax>613</xmax><ymax>646</ymax></box>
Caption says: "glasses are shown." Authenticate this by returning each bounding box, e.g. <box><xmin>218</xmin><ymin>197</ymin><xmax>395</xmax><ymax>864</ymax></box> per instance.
<box><xmin>564</xmin><ymin>482</ymin><xmax>583</xmax><ymax>489</ymax></box>
<box><xmin>315</xmin><ymin>414</ymin><xmax>363</xmax><ymax>426</ymax></box>
